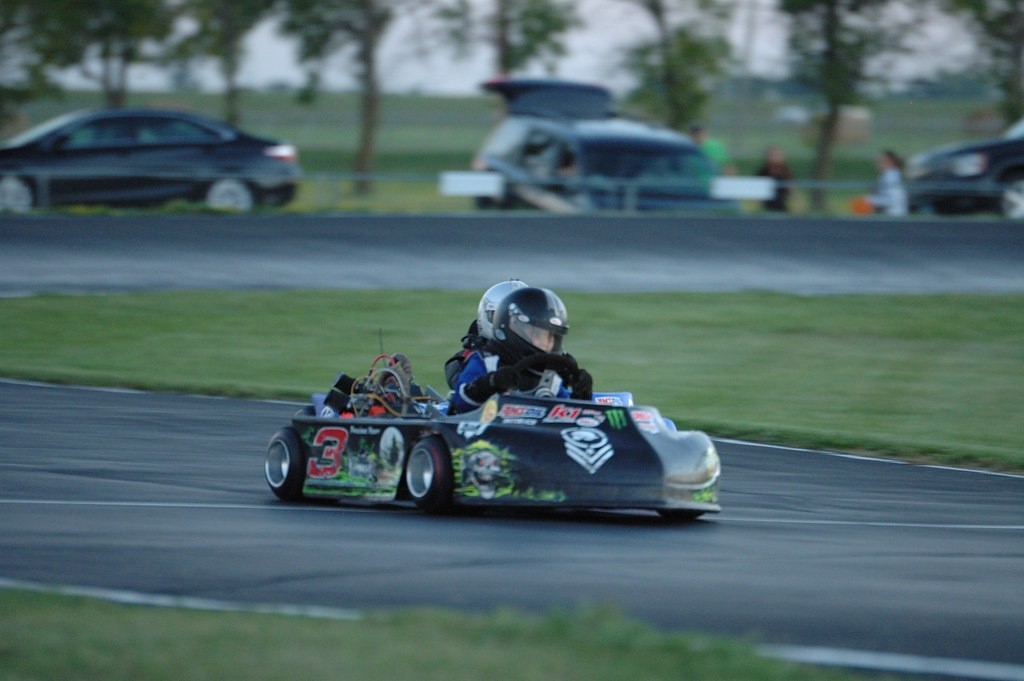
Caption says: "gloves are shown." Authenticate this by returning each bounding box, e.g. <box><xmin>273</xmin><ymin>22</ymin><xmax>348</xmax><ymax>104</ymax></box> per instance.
<box><xmin>491</xmin><ymin>286</ymin><xmax>569</xmax><ymax>358</ymax></box>
<box><xmin>572</xmin><ymin>368</ymin><xmax>593</xmax><ymax>397</ymax></box>
<box><xmin>494</xmin><ymin>365</ymin><xmax>520</xmax><ymax>392</ymax></box>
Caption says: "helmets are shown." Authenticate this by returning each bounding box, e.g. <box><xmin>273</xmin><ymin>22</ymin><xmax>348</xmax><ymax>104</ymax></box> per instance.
<box><xmin>476</xmin><ymin>279</ymin><xmax>529</xmax><ymax>344</ymax></box>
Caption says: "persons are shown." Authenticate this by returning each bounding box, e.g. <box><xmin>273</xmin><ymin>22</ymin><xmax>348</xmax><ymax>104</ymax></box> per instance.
<box><xmin>755</xmin><ymin>145</ymin><xmax>795</xmax><ymax>212</ymax></box>
<box><xmin>690</xmin><ymin>125</ymin><xmax>735</xmax><ymax>175</ymax></box>
<box><xmin>866</xmin><ymin>151</ymin><xmax>908</xmax><ymax>217</ymax></box>
<box><xmin>443</xmin><ymin>278</ymin><xmax>594</xmax><ymax>417</ymax></box>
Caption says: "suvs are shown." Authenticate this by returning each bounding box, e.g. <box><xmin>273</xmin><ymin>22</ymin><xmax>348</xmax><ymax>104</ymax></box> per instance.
<box><xmin>470</xmin><ymin>78</ymin><xmax>743</xmax><ymax>212</ymax></box>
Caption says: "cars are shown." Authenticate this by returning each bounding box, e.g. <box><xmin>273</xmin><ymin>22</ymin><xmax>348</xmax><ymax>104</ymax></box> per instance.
<box><xmin>903</xmin><ymin>117</ymin><xmax>1024</xmax><ymax>220</ymax></box>
<box><xmin>0</xmin><ymin>106</ymin><xmax>300</xmax><ymax>214</ymax></box>
